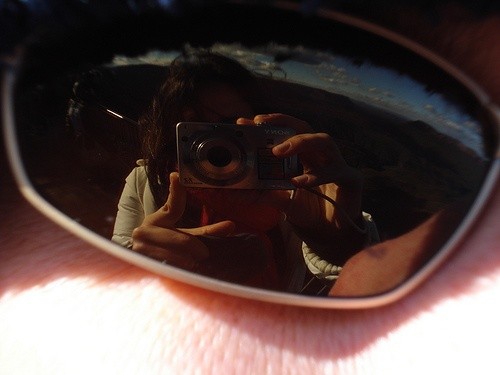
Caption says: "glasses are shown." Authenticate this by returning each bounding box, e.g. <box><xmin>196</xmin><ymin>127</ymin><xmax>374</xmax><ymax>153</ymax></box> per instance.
<box><xmin>177</xmin><ymin>108</ymin><xmax>256</xmax><ymax>133</ymax></box>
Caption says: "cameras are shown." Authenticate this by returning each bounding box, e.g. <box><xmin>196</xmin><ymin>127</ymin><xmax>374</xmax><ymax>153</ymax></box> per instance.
<box><xmin>176</xmin><ymin>122</ymin><xmax>301</xmax><ymax>189</ymax></box>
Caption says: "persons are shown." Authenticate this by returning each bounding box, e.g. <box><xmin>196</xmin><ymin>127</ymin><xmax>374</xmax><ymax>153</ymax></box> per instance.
<box><xmin>109</xmin><ymin>49</ymin><xmax>383</xmax><ymax>298</ymax></box>
